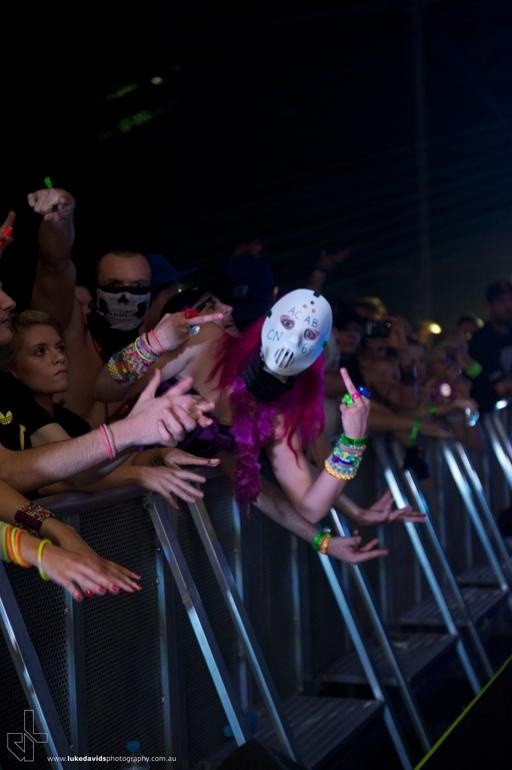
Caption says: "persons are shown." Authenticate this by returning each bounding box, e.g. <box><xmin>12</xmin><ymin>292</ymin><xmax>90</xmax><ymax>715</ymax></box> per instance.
<box><xmin>2</xmin><ymin>183</ymin><xmax>512</xmax><ymax>620</ymax></box>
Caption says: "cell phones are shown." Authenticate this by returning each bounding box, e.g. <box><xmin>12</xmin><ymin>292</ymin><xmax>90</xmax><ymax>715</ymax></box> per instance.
<box><xmin>194</xmin><ymin>297</ymin><xmax>211</xmax><ymax>314</ymax></box>
<box><xmin>365</xmin><ymin>319</ymin><xmax>394</xmax><ymax>338</ymax></box>
